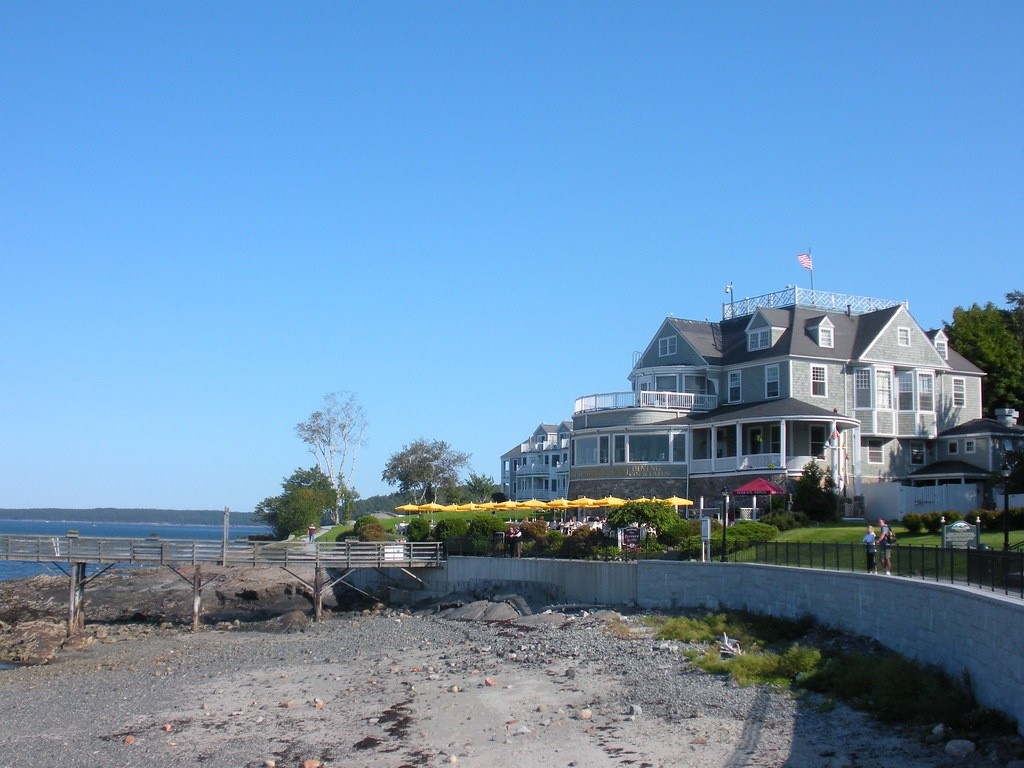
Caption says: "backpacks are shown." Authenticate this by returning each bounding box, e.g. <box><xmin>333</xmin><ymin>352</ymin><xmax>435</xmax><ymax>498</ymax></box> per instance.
<box><xmin>882</xmin><ymin>525</ymin><xmax>897</xmax><ymax>544</ymax></box>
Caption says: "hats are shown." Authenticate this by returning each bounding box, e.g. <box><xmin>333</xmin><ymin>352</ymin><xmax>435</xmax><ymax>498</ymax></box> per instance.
<box><xmin>514</xmin><ymin>526</ymin><xmax>520</xmax><ymax>529</ymax></box>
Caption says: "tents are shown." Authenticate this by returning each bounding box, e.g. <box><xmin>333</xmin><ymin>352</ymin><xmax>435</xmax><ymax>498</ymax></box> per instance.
<box><xmin>733</xmin><ymin>477</ymin><xmax>787</xmax><ymax>521</ymax></box>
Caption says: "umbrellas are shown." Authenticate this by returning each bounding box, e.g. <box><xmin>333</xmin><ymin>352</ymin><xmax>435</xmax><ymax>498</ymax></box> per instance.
<box><xmin>394</xmin><ymin>495</ymin><xmax>693</xmax><ymax>521</ymax></box>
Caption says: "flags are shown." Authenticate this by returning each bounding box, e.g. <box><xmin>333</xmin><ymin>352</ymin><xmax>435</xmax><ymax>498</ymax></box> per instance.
<box><xmin>797</xmin><ymin>254</ymin><xmax>812</xmax><ymax>271</ymax></box>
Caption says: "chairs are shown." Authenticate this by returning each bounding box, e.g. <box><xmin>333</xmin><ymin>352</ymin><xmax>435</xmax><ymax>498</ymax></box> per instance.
<box><xmin>394</xmin><ymin>523</ymin><xmax>406</xmax><ymax>535</ymax></box>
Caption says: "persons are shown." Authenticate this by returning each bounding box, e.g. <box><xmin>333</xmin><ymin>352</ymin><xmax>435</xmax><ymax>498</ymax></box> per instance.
<box><xmin>877</xmin><ymin>518</ymin><xmax>891</xmax><ymax>575</ymax></box>
<box><xmin>863</xmin><ymin>526</ymin><xmax>876</xmax><ymax>574</ymax></box>
<box><xmin>510</xmin><ymin>515</ymin><xmax>604</xmax><ymax>538</ymax></box>
<box><xmin>307</xmin><ymin>523</ymin><xmax>315</xmax><ymax>542</ymax></box>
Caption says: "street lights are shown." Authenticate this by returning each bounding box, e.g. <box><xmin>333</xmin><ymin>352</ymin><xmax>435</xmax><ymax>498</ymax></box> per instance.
<box><xmin>1001</xmin><ymin>461</ymin><xmax>1013</xmax><ymax>552</ymax></box>
<box><xmin>720</xmin><ymin>485</ymin><xmax>729</xmax><ymax>561</ymax></box>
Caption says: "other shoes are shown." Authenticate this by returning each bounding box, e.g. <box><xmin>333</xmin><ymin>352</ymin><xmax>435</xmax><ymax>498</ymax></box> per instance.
<box><xmin>886</xmin><ymin>571</ymin><xmax>891</xmax><ymax>576</ymax></box>
